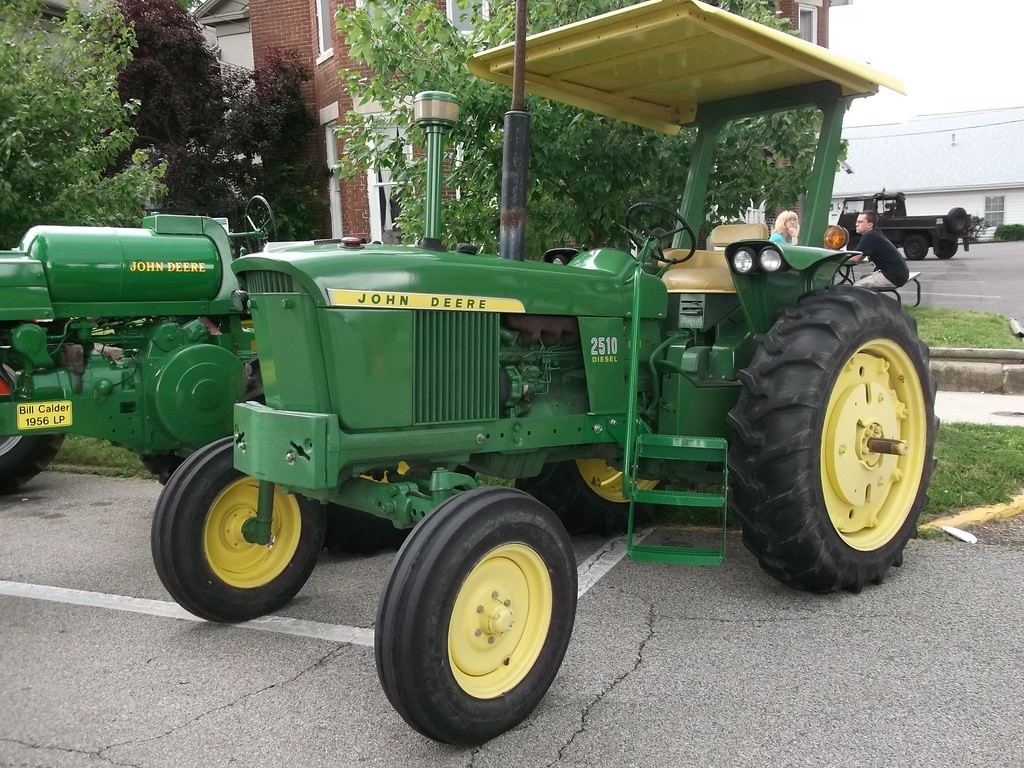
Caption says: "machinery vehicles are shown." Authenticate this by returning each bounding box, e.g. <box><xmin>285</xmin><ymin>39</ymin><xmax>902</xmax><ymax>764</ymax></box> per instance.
<box><xmin>0</xmin><ymin>91</ymin><xmax>493</xmax><ymax>551</ymax></box>
<box><xmin>150</xmin><ymin>1</ymin><xmax>941</xmax><ymax>752</ymax></box>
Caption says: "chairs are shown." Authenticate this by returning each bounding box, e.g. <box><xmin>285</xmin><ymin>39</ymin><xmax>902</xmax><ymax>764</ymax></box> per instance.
<box><xmin>657</xmin><ymin>223</ymin><xmax>768</xmax><ymax>293</ymax></box>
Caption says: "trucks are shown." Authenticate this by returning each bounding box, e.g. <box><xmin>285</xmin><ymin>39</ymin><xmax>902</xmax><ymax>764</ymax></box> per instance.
<box><xmin>836</xmin><ymin>191</ymin><xmax>972</xmax><ymax>261</ymax></box>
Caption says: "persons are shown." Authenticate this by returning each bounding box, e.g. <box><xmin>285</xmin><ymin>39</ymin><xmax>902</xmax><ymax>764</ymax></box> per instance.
<box><xmin>850</xmin><ymin>210</ymin><xmax>909</xmax><ymax>289</ymax></box>
<box><xmin>769</xmin><ymin>210</ymin><xmax>800</xmax><ymax>245</ymax></box>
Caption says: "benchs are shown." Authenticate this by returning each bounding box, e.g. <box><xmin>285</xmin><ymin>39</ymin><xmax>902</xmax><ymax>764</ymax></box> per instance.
<box><xmin>876</xmin><ymin>272</ymin><xmax>921</xmax><ymax>311</ymax></box>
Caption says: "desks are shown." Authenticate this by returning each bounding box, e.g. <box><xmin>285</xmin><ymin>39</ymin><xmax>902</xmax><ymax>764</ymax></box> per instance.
<box><xmin>836</xmin><ymin>251</ymin><xmax>863</xmax><ymax>286</ymax></box>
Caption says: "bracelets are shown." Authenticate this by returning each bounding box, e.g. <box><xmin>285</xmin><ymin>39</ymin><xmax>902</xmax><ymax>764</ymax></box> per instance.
<box><xmin>792</xmin><ymin>235</ymin><xmax>797</xmax><ymax>237</ymax></box>
